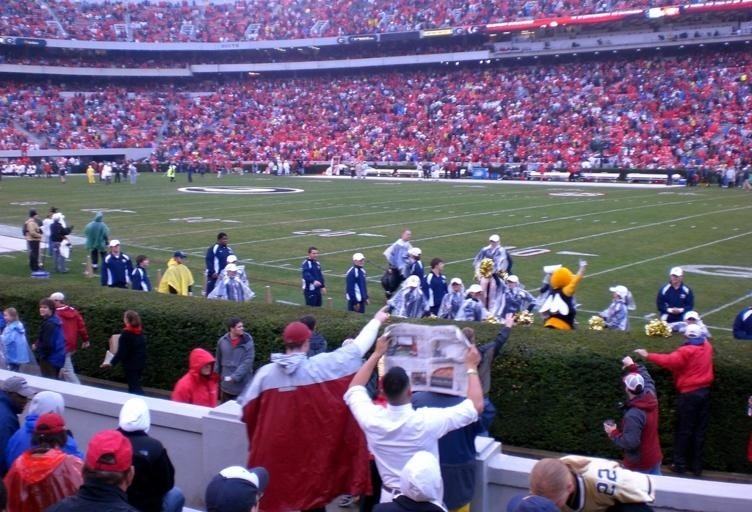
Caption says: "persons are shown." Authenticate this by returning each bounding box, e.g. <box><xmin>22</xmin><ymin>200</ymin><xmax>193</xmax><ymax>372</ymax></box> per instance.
<box><xmin>299</xmin><ymin>246</ymin><xmax>327</xmax><ymax>306</ymax></box>
<box><xmin>634</xmin><ymin>311</ymin><xmax>714</xmax><ymax>478</ymax></box>
<box><xmin>0</xmin><ymin>377</ymin><xmax>186</xmax><ymax>512</ymax></box>
<box><xmin>655</xmin><ymin>266</ymin><xmax>694</xmax><ymax>323</ymax></box>
<box><xmin>0</xmin><ymin>293</ymin><xmax>89</xmax><ymax>384</ymax></box>
<box><xmin>344</xmin><ymin>251</ymin><xmax>371</xmax><ymax>313</ymax></box>
<box><xmin>242</xmin><ymin>304</ymin><xmax>517</xmax><ymax>511</ymax></box>
<box><xmin>174</xmin><ymin>317</ymin><xmax>254</xmax><ymax>408</ymax></box>
<box><xmin>506</xmin><ymin>455</ymin><xmax>655</xmax><ymax>512</ymax></box>
<box><xmin>604</xmin><ymin>356</ymin><xmax>662</xmax><ymax>474</ymax></box>
<box><xmin>596</xmin><ymin>284</ymin><xmax>636</xmax><ymax>331</ymax></box>
<box><xmin>0</xmin><ymin>1</ymin><xmax>751</xmax><ymax>185</ymax></box>
<box><xmin>108</xmin><ymin>310</ymin><xmax>146</xmax><ymax>395</ymax></box>
<box><xmin>22</xmin><ymin>206</ymin><xmax>257</xmax><ymax>303</ymax></box>
<box><xmin>733</xmin><ymin>307</ymin><xmax>752</xmax><ymax>339</ymax></box>
<box><xmin>381</xmin><ymin>228</ymin><xmax>588</xmax><ymax>307</ymax></box>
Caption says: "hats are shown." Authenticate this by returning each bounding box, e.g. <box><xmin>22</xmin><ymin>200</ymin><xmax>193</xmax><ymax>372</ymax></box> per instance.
<box><xmin>85</xmin><ymin>430</ymin><xmax>132</xmax><ymax>471</ymax></box>
<box><xmin>33</xmin><ymin>413</ymin><xmax>66</xmax><ymax>434</ymax></box>
<box><xmin>609</xmin><ymin>285</ymin><xmax>628</xmax><ymax>299</ymax></box>
<box><xmin>505</xmin><ymin>494</ymin><xmax>562</xmax><ymax>511</ymax></box>
<box><xmin>1</xmin><ymin>375</ymin><xmax>37</xmax><ymax>397</ymax></box>
<box><xmin>352</xmin><ymin>253</ymin><xmax>366</xmax><ymax>261</ymax></box>
<box><xmin>669</xmin><ymin>266</ymin><xmax>683</xmax><ymax>277</ymax></box>
<box><xmin>109</xmin><ymin>240</ymin><xmax>121</xmax><ymax>247</ymax></box>
<box><xmin>46</xmin><ymin>292</ymin><xmax>65</xmax><ymax>301</ymax></box>
<box><xmin>29</xmin><ymin>206</ymin><xmax>65</xmax><ymax>222</ymax></box>
<box><xmin>173</xmin><ymin>250</ymin><xmax>187</xmax><ymax>258</ymax></box>
<box><xmin>407</xmin><ymin>234</ymin><xmax>520</xmax><ymax>291</ymax></box>
<box><xmin>684</xmin><ymin>310</ymin><xmax>701</xmax><ymax>338</ymax></box>
<box><xmin>283</xmin><ymin>321</ymin><xmax>312</xmax><ymax>345</ymax></box>
<box><xmin>551</xmin><ymin>267</ymin><xmax>577</xmax><ymax>289</ymax></box>
<box><xmin>225</xmin><ymin>254</ymin><xmax>239</xmax><ymax>272</ymax></box>
<box><xmin>135</xmin><ymin>255</ymin><xmax>147</xmax><ymax>267</ymax></box>
<box><xmin>622</xmin><ymin>372</ymin><xmax>644</xmax><ymax>391</ymax></box>
